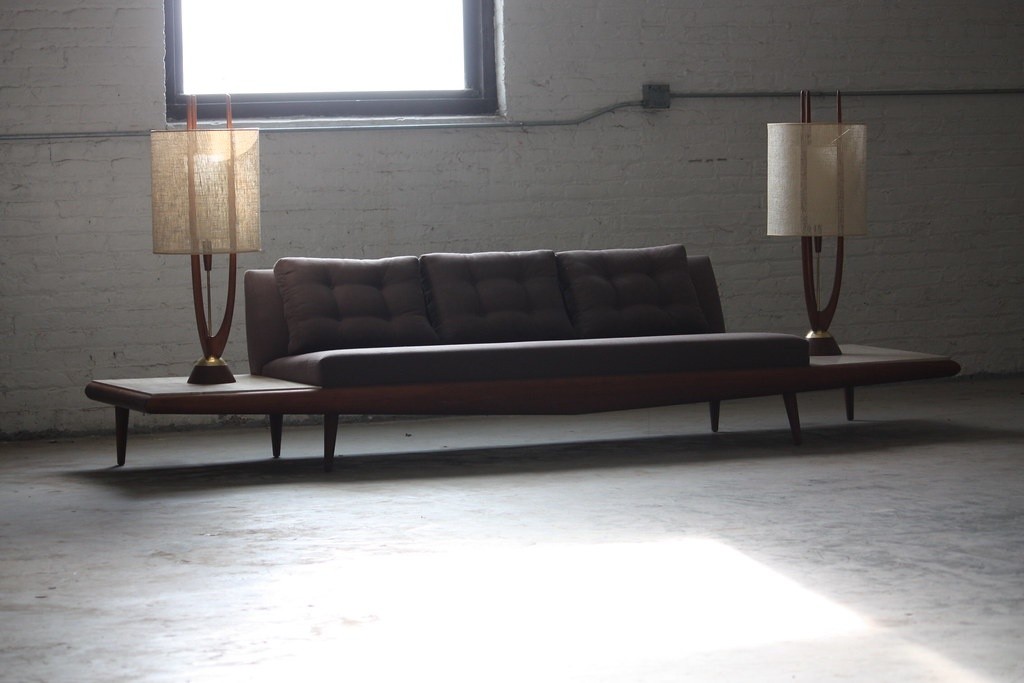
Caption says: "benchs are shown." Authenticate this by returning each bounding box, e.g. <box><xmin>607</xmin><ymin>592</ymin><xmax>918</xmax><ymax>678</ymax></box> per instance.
<box><xmin>242</xmin><ymin>244</ymin><xmax>812</xmax><ymax>471</ymax></box>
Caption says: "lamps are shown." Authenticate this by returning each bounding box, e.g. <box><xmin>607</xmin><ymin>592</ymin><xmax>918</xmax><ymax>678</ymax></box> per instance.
<box><xmin>765</xmin><ymin>91</ymin><xmax>867</xmax><ymax>356</ymax></box>
<box><xmin>148</xmin><ymin>95</ymin><xmax>260</xmax><ymax>386</ymax></box>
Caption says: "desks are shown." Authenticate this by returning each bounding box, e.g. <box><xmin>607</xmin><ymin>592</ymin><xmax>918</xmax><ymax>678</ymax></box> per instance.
<box><xmin>806</xmin><ymin>345</ymin><xmax>962</xmax><ymax>420</ymax></box>
<box><xmin>85</xmin><ymin>373</ymin><xmax>322</xmax><ymax>466</ymax></box>
